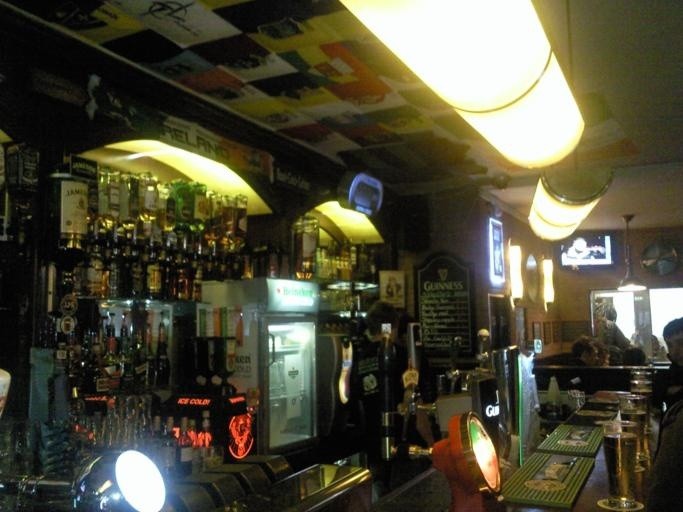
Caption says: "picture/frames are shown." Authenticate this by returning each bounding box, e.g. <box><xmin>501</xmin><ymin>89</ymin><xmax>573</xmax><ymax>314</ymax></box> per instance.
<box><xmin>532</xmin><ymin>320</ymin><xmax>560</xmax><ymax>355</ymax></box>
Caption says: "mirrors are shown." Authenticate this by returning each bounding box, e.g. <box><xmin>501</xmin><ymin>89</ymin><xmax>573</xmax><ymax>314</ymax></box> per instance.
<box><xmin>525</xmin><ymin>252</ymin><xmax>539</xmax><ymax>304</ymax></box>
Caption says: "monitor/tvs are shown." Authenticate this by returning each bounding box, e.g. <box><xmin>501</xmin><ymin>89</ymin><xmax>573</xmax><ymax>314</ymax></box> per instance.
<box><xmin>556</xmin><ymin>231</ymin><xmax>616</xmax><ymax>270</ymax></box>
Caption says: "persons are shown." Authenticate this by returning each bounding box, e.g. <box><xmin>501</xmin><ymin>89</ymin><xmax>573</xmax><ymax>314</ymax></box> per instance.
<box><xmin>564</xmin><ymin>298</ymin><xmax>683</xmax><ymax>512</ymax></box>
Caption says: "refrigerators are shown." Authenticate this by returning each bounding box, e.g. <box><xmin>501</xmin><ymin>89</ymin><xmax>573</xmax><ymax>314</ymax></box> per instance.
<box><xmin>200</xmin><ymin>278</ymin><xmax>318</xmax><ymax>469</ymax></box>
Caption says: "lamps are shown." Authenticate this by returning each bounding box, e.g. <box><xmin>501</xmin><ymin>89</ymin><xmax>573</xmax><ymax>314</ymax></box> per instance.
<box><xmin>508</xmin><ymin>238</ymin><xmax>523</xmax><ymax>305</ymax></box>
<box><xmin>337</xmin><ymin>0</ymin><xmax>585</xmax><ymax>168</ymax></box>
<box><xmin>69</xmin><ymin>450</ymin><xmax>166</xmax><ymax>512</ymax></box>
<box><xmin>541</xmin><ymin>252</ymin><xmax>555</xmax><ymax>310</ymax></box>
<box><xmin>526</xmin><ymin>171</ymin><xmax>613</xmax><ymax>242</ymax></box>
<box><xmin>617</xmin><ymin>213</ymin><xmax>648</xmax><ymax>293</ymax></box>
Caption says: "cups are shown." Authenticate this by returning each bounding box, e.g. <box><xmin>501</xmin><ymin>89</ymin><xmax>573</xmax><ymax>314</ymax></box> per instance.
<box><xmin>630</xmin><ymin>366</ymin><xmax>653</xmax><ymax>433</ymax></box>
<box><xmin>602</xmin><ymin>420</ymin><xmax>638</xmax><ymax>509</ymax></box>
<box><xmin>620</xmin><ymin>395</ymin><xmax>646</xmax><ymax>466</ymax></box>
<box><xmin>568</xmin><ymin>389</ymin><xmax>585</xmax><ymax>413</ymax></box>
<box><xmin>1</xmin><ymin>418</ymin><xmax>40</xmax><ymax>508</ymax></box>
<box><xmin>319</xmin><ymin>287</ymin><xmax>360</xmax><ymax>311</ymax></box>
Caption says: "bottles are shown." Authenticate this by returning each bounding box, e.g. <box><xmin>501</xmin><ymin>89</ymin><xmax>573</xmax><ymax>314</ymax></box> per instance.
<box><xmin>50</xmin><ymin>311</ymin><xmax>170</xmax><ymax>392</ymax></box>
<box><xmin>72</xmin><ymin>241</ymin><xmax>252</xmax><ymax>304</ymax></box>
<box><xmin>96</xmin><ymin>163</ymin><xmax>250</xmax><ymax>257</ymax></box>
<box><xmin>317</xmin><ymin>237</ymin><xmax>376</xmax><ymax>283</ymax></box>
<box><xmin>320</xmin><ymin>314</ymin><xmax>364</xmax><ymax>332</ymax></box>
<box><xmin>75</xmin><ymin>410</ymin><xmax>223</xmax><ymax>479</ymax></box>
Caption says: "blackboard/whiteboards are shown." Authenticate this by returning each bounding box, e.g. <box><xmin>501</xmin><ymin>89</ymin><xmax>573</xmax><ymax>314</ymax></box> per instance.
<box><xmin>413</xmin><ymin>252</ymin><xmax>475</xmax><ymax>357</ymax></box>
